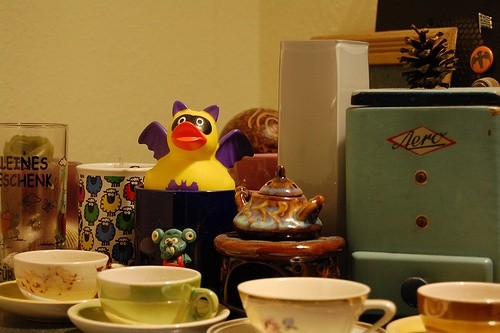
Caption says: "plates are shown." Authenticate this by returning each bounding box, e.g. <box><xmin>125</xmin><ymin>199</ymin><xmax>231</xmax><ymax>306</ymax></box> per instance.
<box><xmin>206</xmin><ymin>317</ymin><xmax>386</xmax><ymax>333</ymax></box>
<box><xmin>384</xmin><ymin>314</ymin><xmax>426</xmax><ymax>333</ymax></box>
<box><xmin>67</xmin><ymin>298</ymin><xmax>230</xmax><ymax>333</ymax></box>
<box><xmin>0</xmin><ymin>281</ymin><xmax>100</xmax><ymax>322</ymax></box>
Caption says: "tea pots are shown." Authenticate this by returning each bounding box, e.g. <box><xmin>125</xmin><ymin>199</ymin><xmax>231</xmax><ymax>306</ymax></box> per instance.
<box><xmin>232</xmin><ymin>166</ymin><xmax>324</xmax><ymax>239</ymax></box>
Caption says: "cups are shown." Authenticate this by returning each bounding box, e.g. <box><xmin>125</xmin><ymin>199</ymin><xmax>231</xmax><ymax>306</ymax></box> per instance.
<box><xmin>237</xmin><ymin>276</ymin><xmax>396</xmax><ymax>333</ymax></box>
<box><xmin>228</xmin><ymin>153</ymin><xmax>278</xmax><ymax>194</ymax></box>
<box><xmin>0</xmin><ymin>122</ymin><xmax>70</xmax><ymax>286</ymax></box>
<box><xmin>134</xmin><ymin>183</ymin><xmax>236</xmax><ymax>302</ymax></box>
<box><xmin>95</xmin><ymin>265</ymin><xmax>220</xmax><ymax>326</ymax></box>
<box><xmin>416</xmin><ymin>281</ymin><xmax>500</xmax><ymax>333</ymax></box>
<box><xmin>74</xmin><ymin>162</ymin><xmax>156</xmax><ymax>267</ymax></box>
<box><xmin>14</xmin><ymin>249</ymin><xmax>111</xmax><ymax>302</ymax></box>
